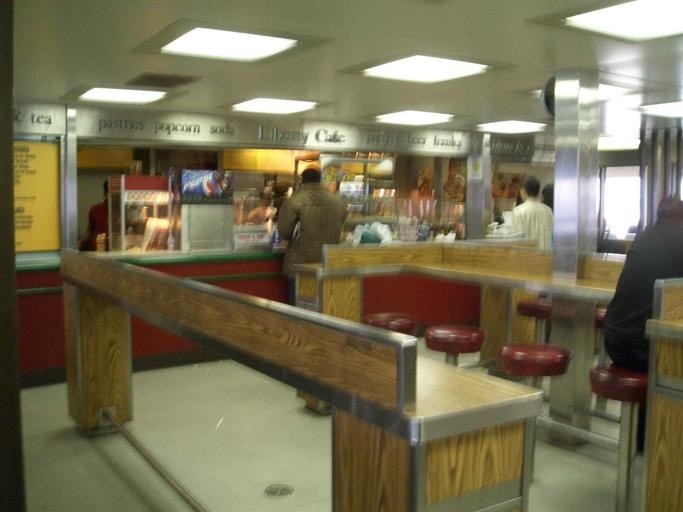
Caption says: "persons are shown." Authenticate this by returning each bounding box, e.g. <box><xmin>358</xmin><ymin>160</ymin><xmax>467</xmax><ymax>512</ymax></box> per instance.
<box><xmin>511</xmin><ymin>175</ymin><xmax>554</xmax><ymax>249</ymax></box>
<box><xmin>248</xmin><ymin>169</ymin><xmax>346</xmax><ymax>301</ymax></box>
<box><xmin>602</xmin><ymin>198</ymin><xmax>683</xmax><ymax>453</ymax></box>
<box><xmin>80</xmin><ymin>179</ymin><xmax>109</xmax><ymax>251</ymax></box>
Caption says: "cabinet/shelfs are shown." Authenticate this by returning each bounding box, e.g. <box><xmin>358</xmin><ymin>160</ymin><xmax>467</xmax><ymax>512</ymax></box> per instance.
<box><xmin>294</xmin><ymin>152</ymin><xmax>397</xmax><ymax>233</ymax></box>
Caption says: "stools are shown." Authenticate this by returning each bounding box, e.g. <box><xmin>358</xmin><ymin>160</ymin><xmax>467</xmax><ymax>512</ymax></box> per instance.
<box><xmin>363</xmin><ymin>312</ymin><xmax>419</xmax><ymax>337</ymax></box>
<box><xmin>517</xmin><ymin>297</ymin><xmax>551</xmax><ymax>344</ymax></box>
<box><xmin>499</xmin><ymin>343</ymin><xmax>571</xmax><ymax>481</ymax></box>
<box><xmin>588</xmin><ymin>366</ymin><xmax>648</xmax><ymax>511</ymax></box>
<box><xmin>425</xmin><ymin>323</ymin><xmax>484</xmax><ymax>366</ymax></box>
<box><xmin>594</xmin><ymin>307</ymin><xmax>609</xmax><ymax>414</ymax></box>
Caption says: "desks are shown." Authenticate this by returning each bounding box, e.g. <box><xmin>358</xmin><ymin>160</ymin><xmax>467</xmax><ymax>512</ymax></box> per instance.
<box><xmin>56</xmin><ymin>245</ymin><xmax>545</xmax><ymax>512</ymax></box>
<box><xmin>290</xmin><ymin>238</ymin><xmax>683</xmax><ymax>511</ymax></box>
